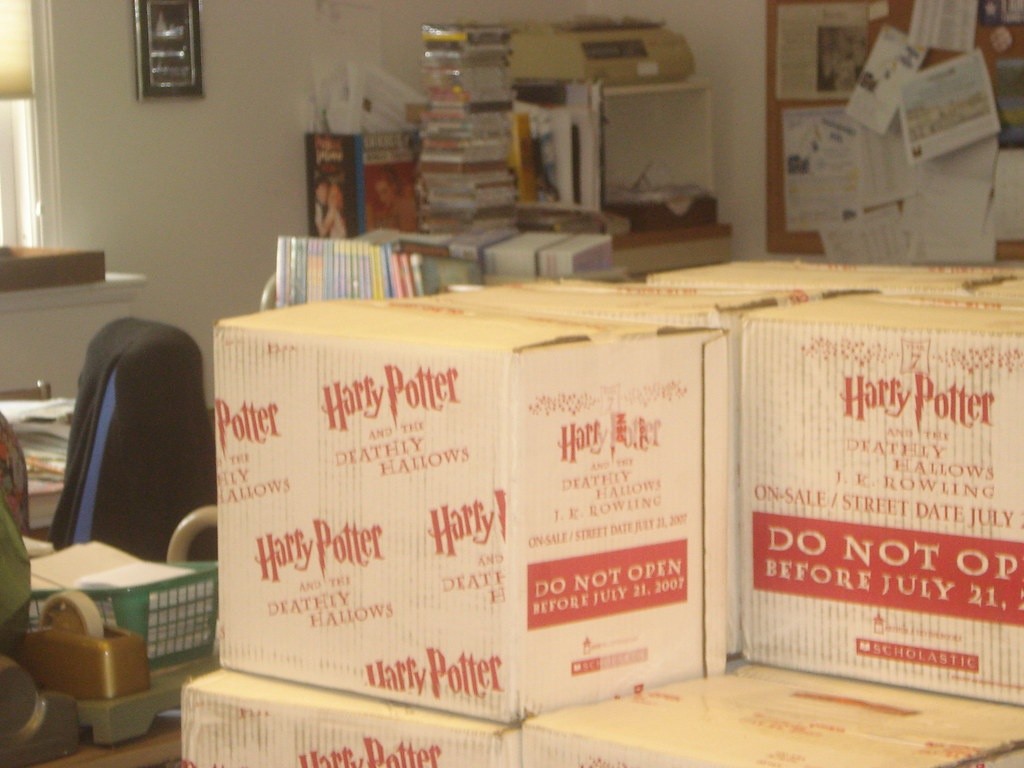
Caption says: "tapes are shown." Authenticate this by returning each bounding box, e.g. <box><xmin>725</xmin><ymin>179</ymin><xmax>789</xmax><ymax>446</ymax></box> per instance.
<box><xmin>39</xmin><ymin>590</ymin><xmax>103</xmax><ymax>638</ymax></box>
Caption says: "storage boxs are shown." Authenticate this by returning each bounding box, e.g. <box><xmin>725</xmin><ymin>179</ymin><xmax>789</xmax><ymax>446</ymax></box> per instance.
<box><xmin>0</xmin><ymin>246</ymin><xmax>106</xmax><ymax>292</ymax></box>
<box><xmin>31</xmin><ymin>563</ymin><xmax>218</xmax><ymax>667</ymax></box>
<box><xmin>603</xmin><ymin>79</ymin><xmax>717</xmax><ymax>202</ymax></box>
<box><xmin>178</xmin><ymin>260</ymin><xmax>1024</xmax><ymax>768</ymax></box>
<box><xmin>303</xmin><ymin>128</ymin><xmax>418</xmax><ymax>241</ymax></box>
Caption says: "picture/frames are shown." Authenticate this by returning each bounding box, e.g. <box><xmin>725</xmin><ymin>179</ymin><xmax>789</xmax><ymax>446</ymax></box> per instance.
<box><xmin>132</xmin><ymin>0</ymin><xmax>206</xmax><ymax>103</ymax></box>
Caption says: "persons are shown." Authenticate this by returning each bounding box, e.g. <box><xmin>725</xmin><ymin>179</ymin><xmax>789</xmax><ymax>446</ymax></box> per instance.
<box><xmin>314</xmin><ymin>177</ymin><xmax>359</xmax><ymax>239</ymax></box>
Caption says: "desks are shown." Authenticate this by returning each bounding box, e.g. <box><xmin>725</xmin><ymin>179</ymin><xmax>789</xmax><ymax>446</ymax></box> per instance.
<box><xmin>0</xmin><ymin>272</ymin><xmax>150</xmax><ymax>316</ymax></box>
<box><xmin>612</xmin><ymin>222</ymin><xmax>733</xmax><ymax>273</ymax></box>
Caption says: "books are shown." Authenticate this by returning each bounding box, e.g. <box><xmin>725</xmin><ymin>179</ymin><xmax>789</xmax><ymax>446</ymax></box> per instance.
<box><xmin>304</xmin><ymin>130</ymin><xmax>420</xmax><ymax>240</ymax></box>
<box><xmin>416</xmin><ymin>24</ymin><xmax>609</xmax><ymax>232</ymax></box>
<box><xmin>275</xmin><ymin>227</ymin><xmax>612</xmax><ymax>308</ymax></box>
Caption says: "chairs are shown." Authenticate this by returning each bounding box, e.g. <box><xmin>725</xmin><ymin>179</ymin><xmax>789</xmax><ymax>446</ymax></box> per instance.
<box><xmin>49</xmin><ymin>317</ymin><xmax>216</xmax><ymax>563</ymax></box>
